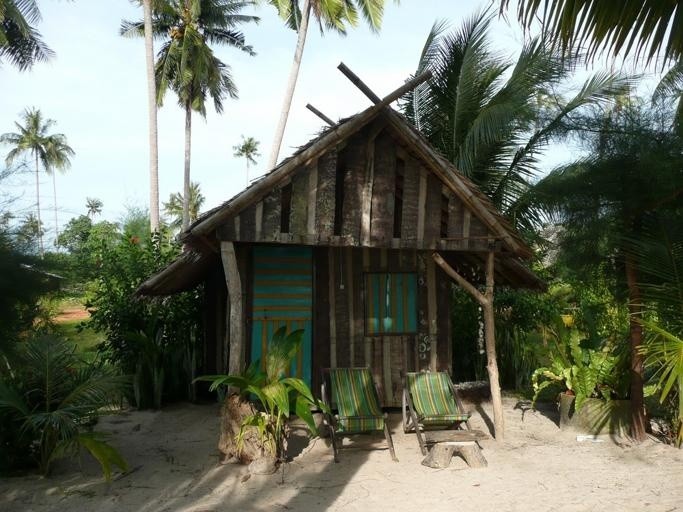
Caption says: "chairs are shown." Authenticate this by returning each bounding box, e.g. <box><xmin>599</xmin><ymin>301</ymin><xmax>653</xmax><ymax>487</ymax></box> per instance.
<box><xmin>399</xmin><ymin>367</ymin><xmax>489</xmax><ymax>468</ymax></box>
<box><xmin>321</xmin><ymin>365</ymin><xmax>397</xmax><ymax>463</ymax></box>
